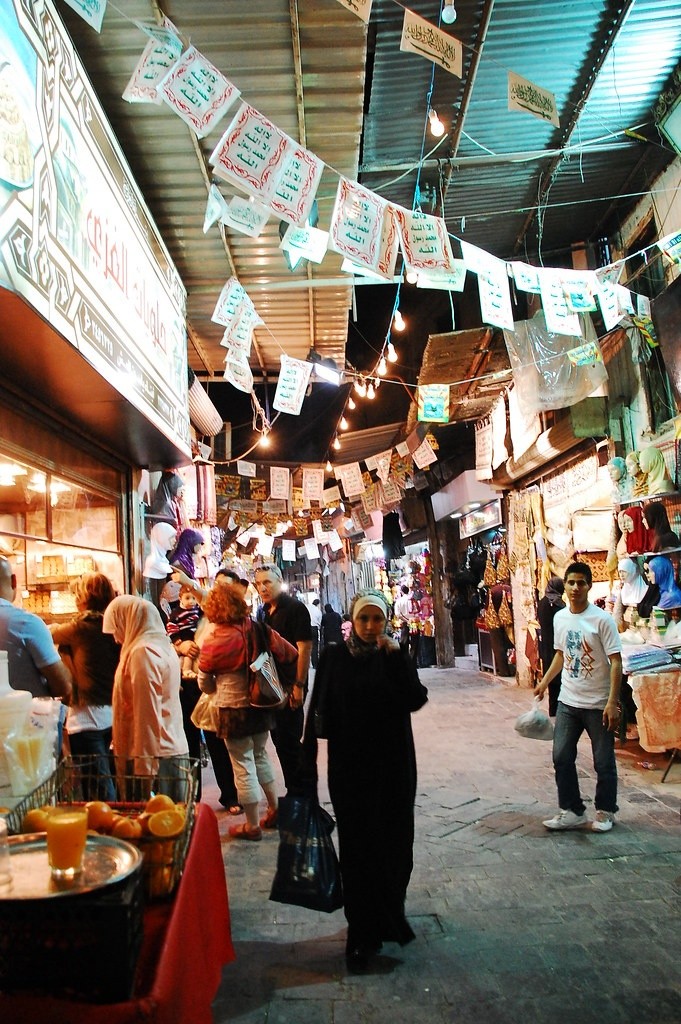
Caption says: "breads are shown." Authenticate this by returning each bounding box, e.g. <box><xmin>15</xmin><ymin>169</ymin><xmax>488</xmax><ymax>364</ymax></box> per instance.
<box><xmin>23</xmin><ymin>591</ymin><xmax>50</xmax><ymax>613</ymax></box>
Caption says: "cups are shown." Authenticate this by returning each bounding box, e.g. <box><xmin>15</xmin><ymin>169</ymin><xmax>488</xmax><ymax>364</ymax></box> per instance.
<box><xmin>42</xmin><ymin>805</ymin><xmax>89</xmax><ymax>875</ymax></box>
<box><xmin>1</xmin><ymin>818</ymin><xmax>17</xmax><ymax>894</ymax></box>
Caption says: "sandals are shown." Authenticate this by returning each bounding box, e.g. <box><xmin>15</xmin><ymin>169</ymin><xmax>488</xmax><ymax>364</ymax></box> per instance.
<box><xmin>226</xmin><ymin>806</ymin><xmax>243</xmax><ymax>815</ymax></box>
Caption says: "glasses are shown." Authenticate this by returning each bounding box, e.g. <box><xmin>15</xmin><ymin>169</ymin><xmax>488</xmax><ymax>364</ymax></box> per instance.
<box><xmin>643</xmin><ymin>568</ymin><xmax>649</xmax><ymax>573</ymax></box>
<box><xmin>255</xmin><ymin>566</ymin><xmax>279</xmax><ymax>579</ymax></box>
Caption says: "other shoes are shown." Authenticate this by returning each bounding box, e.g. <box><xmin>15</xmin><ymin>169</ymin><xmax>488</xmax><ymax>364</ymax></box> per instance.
<box><xmin>230</xmin><ymin>824</ymin><xmax>262</xmax><ymax>842</ymax></box>
<box><xmin>260</xmin><ymin>808</ymin><xmax>279</xmax><ymax>831</ymax></box>
<box><xmin>347</xmin><ymin>938</ymin><xmax>384</xmax><ymax>959</ymax></box>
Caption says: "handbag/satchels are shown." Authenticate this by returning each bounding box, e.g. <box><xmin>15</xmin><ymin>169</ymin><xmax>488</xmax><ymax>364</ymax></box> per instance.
<box><xmin>513</xmin><ymin>694</ymin><xmax>555</xmax><ymax>742</ymax></box>
<box><xmin>247</xmin><ymin>620</ymin><xmax>289</xmax><ymax>710</ymax></box>
<box><xmin>269</xmin><ymin>747</ymin><xmax>345</xmax><ymax>913</ymax></box>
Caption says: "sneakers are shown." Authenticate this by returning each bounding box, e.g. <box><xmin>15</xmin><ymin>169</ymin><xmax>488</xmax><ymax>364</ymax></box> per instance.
<box><xmin>591</xmin><ymin>810</ymin><xmax>615</xmax><ymax>831</ymax></box>
<box><xmin>542</xmin><ymin>811</ymin><xmax>587</xmax><ymax>830</ymax></box>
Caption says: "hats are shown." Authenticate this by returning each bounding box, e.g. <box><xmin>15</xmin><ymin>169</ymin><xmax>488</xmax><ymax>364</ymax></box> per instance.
<box><xmin>353</xmin><ymin>595</ymin><xmax>389</xmax><ymax>625</ymax></box>
<box><xmin>349</xmin><ymin>587</ymin><xmax>390</xmax><ymax>623</ymax></box>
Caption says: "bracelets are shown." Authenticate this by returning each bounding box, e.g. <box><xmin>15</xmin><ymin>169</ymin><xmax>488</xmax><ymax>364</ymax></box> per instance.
<box><xmin>294</xmin><ymin>678</ymin><xmax>305</xmax><ymax>689</ymax></box>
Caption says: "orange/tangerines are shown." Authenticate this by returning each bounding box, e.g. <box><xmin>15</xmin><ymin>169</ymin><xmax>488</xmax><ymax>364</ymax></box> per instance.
<box><xmin>22</xmin><ymin>794</ymin><xmax>188</xmax><ymax>896</ymax></box>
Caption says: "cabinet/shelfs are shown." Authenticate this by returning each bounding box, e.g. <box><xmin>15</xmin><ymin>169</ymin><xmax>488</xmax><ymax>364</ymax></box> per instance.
<box><xmin>618</xmin><ymin>488</ymin><xmax>681</xmax><ymax>652</ymax></box>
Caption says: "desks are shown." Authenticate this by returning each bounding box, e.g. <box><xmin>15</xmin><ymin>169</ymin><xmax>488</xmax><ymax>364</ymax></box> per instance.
<box><xmin>0</xmin><ymin>798</ymin><xmax>234</xmax><ymax>1024</ymax></box>
<box><xmin>618</xmin><ymin>665</ymin><xmax>681</xmax><ymax>783</ymax></box>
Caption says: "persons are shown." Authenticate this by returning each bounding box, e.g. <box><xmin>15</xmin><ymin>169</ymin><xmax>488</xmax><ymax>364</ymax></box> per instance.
<box><xmin>167</xmin><ymin>570</ymin><xmax>250</xmax><ymax>814</ymax></box>
<box><xmin>394</xmin><ymin>585</ymin><xmax>412</xmax><ymax>653</ymax></box>
<box><xmin>533</xmin><ymin>563</ymin><xmax>622</xmax><ymax>832</ymax></box>
<box><xmin>308</xmin><ymin>599</ymin><xmax>322</xmax><ymax>668</ymax></box>
<box><xmin>151</xmin><ymin>468</ymin><xmax>182</xmax><ymax>517</ymax></box>
<box><xmin>321</xmin><ymin>604</ymin><xmax>341</xmax><ymax>646</ymax></box>
<box><xmin>198</xmin><ymin>582</ymin><xmax>298</xmax><ymax>841</ymax></box>
<box><xmin>602</xmin><ymin>447</ymin><xmax>681</xmax><ymax>611</ymax></box>
<box><xmin>314</xmin><ymin>588</ymin><xmax>429</xmax><ymax>969</ymax></box>
<box><xmin>254</xmin><ymin>564</ymin><xmax>314</xmax><ymax>811</ymax></box>
<box><xmin>539</xmin><ymin>577</ymin><xmax>568</xmax><ymax>719</ymax></box>
<box><xmin>143</xmin><ymin>522</ymin><xmax>173</xmax><ymax>580</ymax></box>
<box><xmin>0</xmin><ymin>557</ymin><xmax>72</xmax><ymax>705</ymax></box>
<box><xmin>46</xmin><ymin>571</ymin><xmax>120</xmax><ymax>800</ymax></box>
<box><xmin>103</xmin><ymin>595</ymin><xmax>191</xmax><ymax>805</ymax></box>
<box><xmin>171</xmin><ymin>527</ymin><xmax>204</xmax><ymax>580</ymax></box>
<box><xmin>341</xmin><ymin>613</ymin><xmax>352</xmax><ymax>642</ymax></box>
